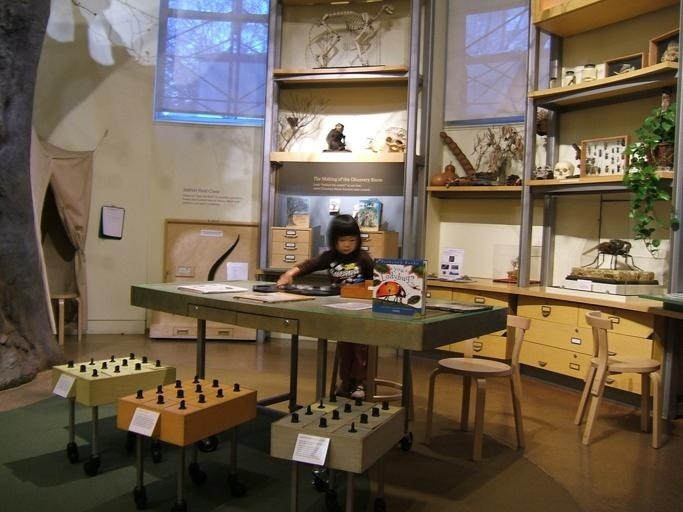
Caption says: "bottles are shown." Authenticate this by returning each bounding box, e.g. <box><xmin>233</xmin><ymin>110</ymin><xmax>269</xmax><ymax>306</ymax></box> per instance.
<box><xmin>549</xmin><ymin>63</ymin><xmax>598</xmax><ymax>88</ymax></box>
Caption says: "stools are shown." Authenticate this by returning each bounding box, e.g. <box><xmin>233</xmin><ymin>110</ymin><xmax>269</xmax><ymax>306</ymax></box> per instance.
<box><xmin>50</xmin><ymin>290</ymin><xmax>82</xmax><ymax>346</ymax></box>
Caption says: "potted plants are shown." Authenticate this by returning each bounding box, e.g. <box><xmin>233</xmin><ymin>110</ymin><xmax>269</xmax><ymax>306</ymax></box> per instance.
<box><xmin>620</xmin><ymin>102</ymin><xmax>682</xmax><ymax>260</ymax></box>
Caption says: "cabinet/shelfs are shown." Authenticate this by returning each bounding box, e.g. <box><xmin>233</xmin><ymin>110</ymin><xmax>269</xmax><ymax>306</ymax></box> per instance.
<box><xmin>510</xmin><ymin>0</ymin><xmax>681</xmax><ymax>410</ymax></box>
<box><xmin>254</xmin><ymin>1</ymin><xmax>435</xmax><ymax>346</ymax></box>
<box><xmin>423</xmin><ymin>179</ymin><xmax>523</xmax><ymax>364</ymax></box>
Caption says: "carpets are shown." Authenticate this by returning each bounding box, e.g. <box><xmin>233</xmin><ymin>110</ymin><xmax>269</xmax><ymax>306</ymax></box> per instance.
<box><xmin>1</xmin><ymin>392</ymin><xmax>580</xmax><ymax>512</ymax></box>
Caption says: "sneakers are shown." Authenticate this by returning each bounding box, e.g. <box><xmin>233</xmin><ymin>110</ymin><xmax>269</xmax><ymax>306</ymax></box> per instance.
<box><xmin>335</xmin><ymin>384</ymin><xmax>366</xmax><ymax>399</ymax></box>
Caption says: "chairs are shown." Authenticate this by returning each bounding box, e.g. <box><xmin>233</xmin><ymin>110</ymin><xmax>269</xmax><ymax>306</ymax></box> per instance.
<box><xmin>426</xmin><ymin>313</ymin><xmax>532</xmax><ymax>461</ymax></box>
<box><xmin>574</xmin><ymin>308</ymin><xmax>664</xmax><ymax>449</ymax></box>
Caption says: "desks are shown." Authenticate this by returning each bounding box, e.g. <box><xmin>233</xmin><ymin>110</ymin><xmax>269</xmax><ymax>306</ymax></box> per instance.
<box><xmin>127</xmin><ymin>279</ymin><xmax>515</xmax><ymax>450</ymax></box>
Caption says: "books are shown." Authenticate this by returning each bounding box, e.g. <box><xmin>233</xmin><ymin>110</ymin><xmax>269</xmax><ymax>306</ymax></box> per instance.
<box><xmin>370</xmin><ymin>258</ymin><xmax>427</xmax><ymax>318</ymax></box>
<box><xmin>424</xmin><ymin>300</ymin><xmax>495</xmax><ymax>314</ymax></box>
<box><xmin>174</xmin><ymin>282</ymin><xmax>249</xmax><ymax>294</ymax></box>
<box><xmin>232</xmin><ymin>292</ymin><xmax>316</xmax><ymax>306</ymax></box>
<box><xmin>356</xmin><ymin>198</ymin><xmax>383</xmax><ymax>233</ymax></box>
<box><xmin>285</xmin><ymin>196</ymin><xmax>310</xmax><ymax>227</ymax></box>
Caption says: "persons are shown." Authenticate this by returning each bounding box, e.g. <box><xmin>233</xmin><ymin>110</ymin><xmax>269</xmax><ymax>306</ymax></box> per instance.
<box><xmin>275</xmin><ymin>214</ymin><xmax>374</xmax><ymax>402</ymax></box>
